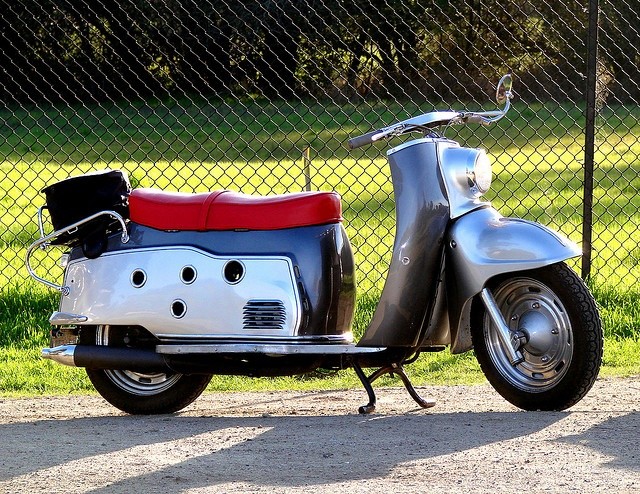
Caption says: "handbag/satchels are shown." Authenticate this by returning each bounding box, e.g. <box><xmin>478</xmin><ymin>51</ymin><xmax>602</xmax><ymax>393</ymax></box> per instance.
<box><xmin>40</xmin><ymin>168</ymin><xmax>131</xmax><ymax>258</ymax></box>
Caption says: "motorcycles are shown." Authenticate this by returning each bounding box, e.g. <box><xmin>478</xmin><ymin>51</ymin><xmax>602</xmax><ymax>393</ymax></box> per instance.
<box><xmin>26</xmin><ymin>74</ymin><xmax>603</xmax><ymax>415</ymax></box>
